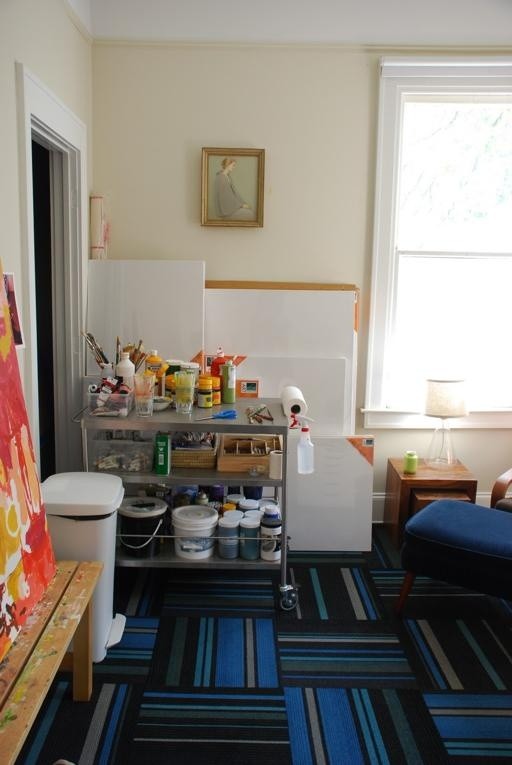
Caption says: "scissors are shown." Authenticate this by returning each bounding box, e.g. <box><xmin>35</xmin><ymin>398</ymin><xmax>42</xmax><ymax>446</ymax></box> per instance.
<box><xmin>193</xmin><ymin>409</ymin><xmax>237</xmax><ymax>421</ymax></box>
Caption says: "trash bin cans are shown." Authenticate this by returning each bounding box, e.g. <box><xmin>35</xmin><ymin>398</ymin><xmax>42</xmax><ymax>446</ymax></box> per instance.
<box><xmin>41</xmin><ymin>472</ymin><xmax>127</xmax><ymax>666</ymax></box>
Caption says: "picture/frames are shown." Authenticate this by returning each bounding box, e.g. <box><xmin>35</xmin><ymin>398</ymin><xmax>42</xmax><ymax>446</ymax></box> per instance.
<box><xmin>202</xmin><ymin>147</ymin><xmax>267</xmax><ymax>227</ymax></box>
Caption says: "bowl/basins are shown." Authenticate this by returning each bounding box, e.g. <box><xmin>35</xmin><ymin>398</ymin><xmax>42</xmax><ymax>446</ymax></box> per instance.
<box><xmin>153</xmin><ymin>393</ymin><xmax>172</xmax><ymax>412</ymax></box>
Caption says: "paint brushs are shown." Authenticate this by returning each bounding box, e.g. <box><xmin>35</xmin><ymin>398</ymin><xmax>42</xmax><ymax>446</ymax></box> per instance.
<box><xmin>246</xmin><ymin>406</ymin><xmax>273</xmax><ymax>424</ymax></box>
<box><xmin>80</xmin><ymin>330</ymin><xmax>149</xmax><ymax>373</ymax></box>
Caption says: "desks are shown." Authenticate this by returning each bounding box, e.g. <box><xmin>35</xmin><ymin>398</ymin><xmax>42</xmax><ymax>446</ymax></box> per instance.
<box><xmin>0</xmin><ymin>561</ymin><xmax>99</xmax><ymax>764</ymax></box>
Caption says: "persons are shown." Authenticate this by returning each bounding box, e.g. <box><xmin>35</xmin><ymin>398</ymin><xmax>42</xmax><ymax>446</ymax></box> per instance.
<box><xmin>215</xmin><ymin>158</ymin><xmax>254</xmax><ymax>218</ymax></box>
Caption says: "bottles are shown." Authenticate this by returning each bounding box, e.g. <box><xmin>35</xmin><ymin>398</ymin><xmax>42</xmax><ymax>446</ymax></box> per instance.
<box><xmin>218</xmin><ymin>493</ymin><xmax>282</xmax><ymax>561</ymax></box>
<box><xmin>145</xmin><ymin>349</ymin><xmax>162</xmax><ymax>376</ymax></box>
<box><xmin>222</xmin><ymin>358</ymin><xmax>236</xmax><ymax>405</ymax></box>
<box><xmin>211</xmin><ymin>346</ymin><xmax>227</xmax><ymax>383</ymax></box>
<box><xmin>403</xmin><ymin>451</ymin><xmax>418</xmax><ymax>473</ymax></box>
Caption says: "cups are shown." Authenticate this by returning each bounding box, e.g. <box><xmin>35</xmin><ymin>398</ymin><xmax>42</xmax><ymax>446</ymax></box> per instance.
<box><xmin>134</xmin><ymin>375</ymin><xmax>154</xmax><ymax>418</ymax></box>
<box><xmin>174</xmin><ymin>370</ymin><xmax>196</xmax><ymax>415</ymax></box>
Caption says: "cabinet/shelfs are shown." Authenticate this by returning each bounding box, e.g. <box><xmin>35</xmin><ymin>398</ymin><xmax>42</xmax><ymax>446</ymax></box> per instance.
<box><xmin>86</xmin><ymin>400</ymin><xmax>299</xmax><ymax>609</ymax></box>
<box><xmin>383</xmin><ymin>456</ymin><xmax>476</xmax><ymax>544</ymax></box>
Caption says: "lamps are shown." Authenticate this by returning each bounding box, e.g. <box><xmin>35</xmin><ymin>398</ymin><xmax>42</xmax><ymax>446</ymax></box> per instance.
<box><xmin>424</xmin><ymin>379</ymin><xmax>469</xmax><ymax>466</ymax></box>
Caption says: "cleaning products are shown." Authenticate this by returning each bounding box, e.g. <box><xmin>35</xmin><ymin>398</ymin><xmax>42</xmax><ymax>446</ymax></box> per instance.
<box><xmin>290</xmin><ymin>411</ymin><xmax>315</xmax><ymax>474</ymax></box>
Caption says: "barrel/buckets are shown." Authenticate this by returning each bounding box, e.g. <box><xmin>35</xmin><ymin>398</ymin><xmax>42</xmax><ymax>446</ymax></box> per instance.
<box><xmin>171</xmin><ymin>504</ymin><xmax>219</xmax><ymax>559</ymax></box>
<box><xmin>118</xmin><ymin>496</ymin><xmax>168</xmax><ymax>558</ymax></box>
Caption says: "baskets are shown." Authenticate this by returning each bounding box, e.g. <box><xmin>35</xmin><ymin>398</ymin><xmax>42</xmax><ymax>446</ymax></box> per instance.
<box><xmin>170</xmin><ymin>433</ymin><xmax>218</xmax><ymax>469</ymax></box>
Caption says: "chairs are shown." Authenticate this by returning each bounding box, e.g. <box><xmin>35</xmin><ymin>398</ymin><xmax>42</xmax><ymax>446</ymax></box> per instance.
<box><xmin>389</xmin><ymin>468</ymin><xmax>510</xmax><ymax>624</ymax></box>
